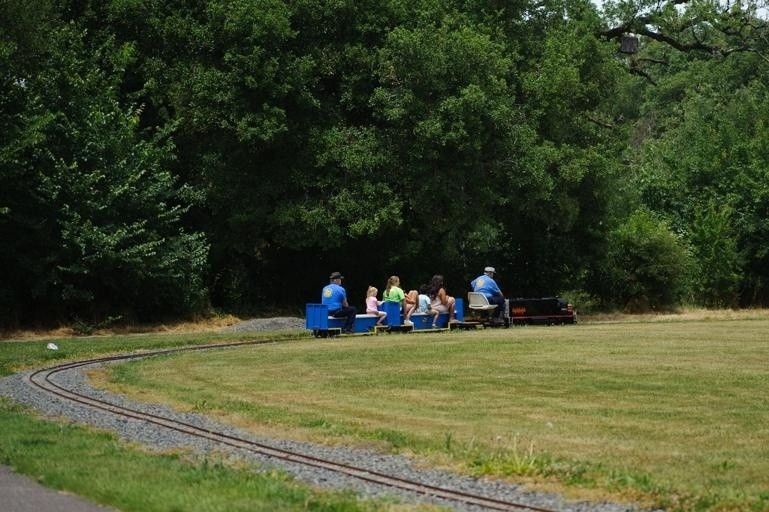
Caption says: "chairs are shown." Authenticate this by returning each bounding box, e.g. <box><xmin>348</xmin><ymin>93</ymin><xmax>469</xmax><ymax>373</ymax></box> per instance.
<box><xmin>467</xmin><ymin>292</ymin><xmax>498</xmax><ymax>320</ymax></box>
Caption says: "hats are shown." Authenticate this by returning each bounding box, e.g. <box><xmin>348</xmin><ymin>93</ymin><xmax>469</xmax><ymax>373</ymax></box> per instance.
<box><xmin>484</xmin><ymin>266</ymin><xmax>496</xmax><ymax>273</ymax></box>
<box><xmin>329</xmin><ymin>271</ymin><xmax>345</xmax><ymax>280</ymax></box>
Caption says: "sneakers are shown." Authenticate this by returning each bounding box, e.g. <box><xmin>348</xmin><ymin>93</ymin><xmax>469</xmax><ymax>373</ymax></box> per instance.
<box><xmin>403</xmin><ymin>319</ymin><xmax>414</xmax><ymax>326</ymax></box>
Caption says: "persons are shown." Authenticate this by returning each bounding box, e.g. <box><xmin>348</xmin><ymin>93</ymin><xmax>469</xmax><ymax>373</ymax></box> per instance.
<box><xmin>428</xmin><ymin>275</ymin><xmax>458</xmax><ymax>322</ymax></box>
<box><xmin>365</xmin><ymin>285</ymin><xmax>388</xmax><ymax>326</ymax></box>
<box><xmin>382</xmin><ymin>276</ymin><xmax>416</xmax><ymax>325</ymax></box>
<box><xmin>418</xmin><ymin>284</ymin><xmax>440</xmax><ymax>329</ymax></box>
<box><xmin>470</xmin><ymin>266</ymin><xmax>505</xmax><ymax>323</ymax></box>
<box><xmin>321</xmin><ymin>271</ymin><xmax>356</xmax><ymax>333</ymax></box>
<box><xmin>402</xmin><ymin>289</ymin><xmax>419</xmax><ymax>313</ymax></box>
